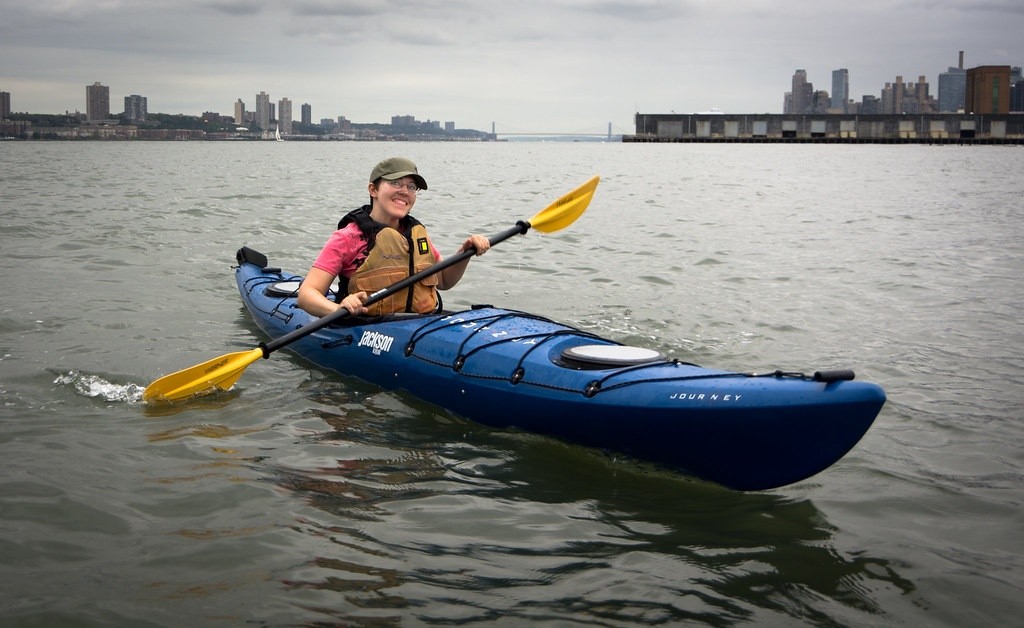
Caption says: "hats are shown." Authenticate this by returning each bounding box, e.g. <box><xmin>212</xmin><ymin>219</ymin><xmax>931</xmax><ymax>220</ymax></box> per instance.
<box><xmin>369</xmin><ymin>157</ymin><xmax>427</xmax><ymax>190</ymax></box>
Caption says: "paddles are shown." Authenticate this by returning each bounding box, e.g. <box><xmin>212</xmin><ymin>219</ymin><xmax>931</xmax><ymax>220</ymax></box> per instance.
<box><xmin>144</xmin><ymin>174</ymin><xmax>598</xmax><ymax>407</ymax></box>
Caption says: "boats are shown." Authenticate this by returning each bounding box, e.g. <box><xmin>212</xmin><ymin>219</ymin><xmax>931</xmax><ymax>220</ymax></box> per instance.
<box><xmin>230</xmin><ymin>245</ymin><xmax>888</xmax><ymax>493</ymax></box>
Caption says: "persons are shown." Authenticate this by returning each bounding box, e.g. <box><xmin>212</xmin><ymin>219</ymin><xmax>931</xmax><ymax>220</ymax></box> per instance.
<box><xmin>297</xmin><ymin>157</ymin><xmax>490</xmax><ymax>319</ymax></box>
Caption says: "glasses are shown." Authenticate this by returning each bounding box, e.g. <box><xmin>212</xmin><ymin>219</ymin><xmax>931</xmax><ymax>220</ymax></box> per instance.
<box><xmin>376</xmin><ymin>178</ymin><xmax>422</xmax><ymax>195</ymax></box>
